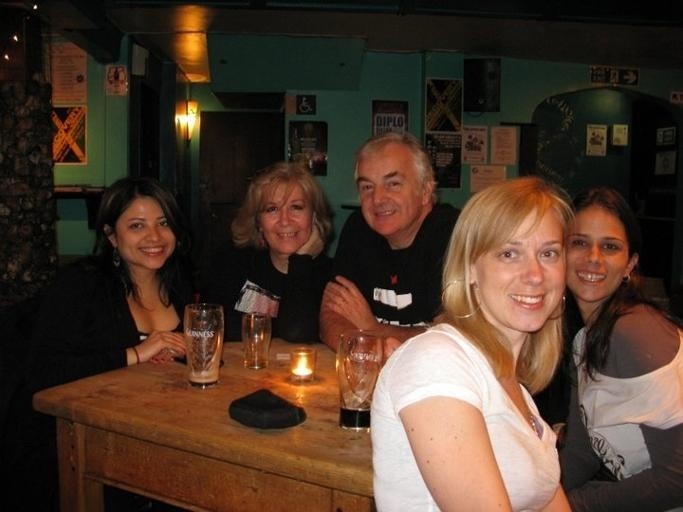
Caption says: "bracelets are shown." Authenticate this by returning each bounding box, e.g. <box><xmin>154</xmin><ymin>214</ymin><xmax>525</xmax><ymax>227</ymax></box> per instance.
<box><xmin>132</xmin><ymin>346</ymin><xmax>141</xmax><ymax>364</ymax></box>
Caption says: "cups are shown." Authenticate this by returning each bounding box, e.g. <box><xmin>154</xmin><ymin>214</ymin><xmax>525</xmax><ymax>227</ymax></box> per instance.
<box><xmin>180</xmin><ymin>301</ymin><xmax>226</xmax><ymax>388</ymax></box>
<box><xmin>240</xmin><ymin>311</ymin><xmax>272</xmax><ymax>371</ymax></box>
<box><xmin>335</xmin><ymin>327</ymin><xmax>381</xmax><ymax>432</ymax></box>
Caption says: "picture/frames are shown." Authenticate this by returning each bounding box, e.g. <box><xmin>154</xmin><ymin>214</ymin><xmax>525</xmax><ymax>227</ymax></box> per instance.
<box><xmin>586</xmin><ymin>123</ymin><xmax>608</xmax><ymax>157</ymax></box>
<box><xmin>52</xmin><ymin>104</ymin><xmax>88</xmax><ymax>166</ymax></box>
<box><xmin>424</xmin><ymin>76</ymin><xmax>464</xmax><ymax>134</ymax></box>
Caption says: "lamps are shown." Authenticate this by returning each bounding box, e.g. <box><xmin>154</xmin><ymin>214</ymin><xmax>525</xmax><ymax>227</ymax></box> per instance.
<box><xmin>173</xmin><ymin>30</ymin><xmax>213</xmax><ymax>84</ymax></box>
<box><xmin>174</xmin><ymin>99</ymin><xmax>199</xmax><ymax>149</ymax></box>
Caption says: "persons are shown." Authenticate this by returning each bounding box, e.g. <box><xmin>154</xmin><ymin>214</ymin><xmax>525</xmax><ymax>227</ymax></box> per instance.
<box><xmin>319</xmin><ymin>128</ymin><xmax>461</xmax><ymax>355</ymax></box>
<box><xmin>70</xmin><ymin>173</ymin><xmax>219</xmax><ymax>369</ymax></box>
<box><xmin>214</xmin><ymin>160</ymin><xmax>339</xmax><ymax>345</ymax></box>
<box><xmin>370</xmin><ymin>175</ymin><xmax>579</xmax><ymax>512</ymax></box>
<box><xmin>553</xmin><ymin>192</ymin><xmax>682</xmax><ymax>511</ymax></box>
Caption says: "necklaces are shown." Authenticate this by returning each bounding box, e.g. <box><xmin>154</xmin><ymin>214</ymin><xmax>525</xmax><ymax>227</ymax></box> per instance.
<box><xmin>529</xmin><ymin>414</ymin><xmax>541</xmax><ymax>437</ymax></box>
